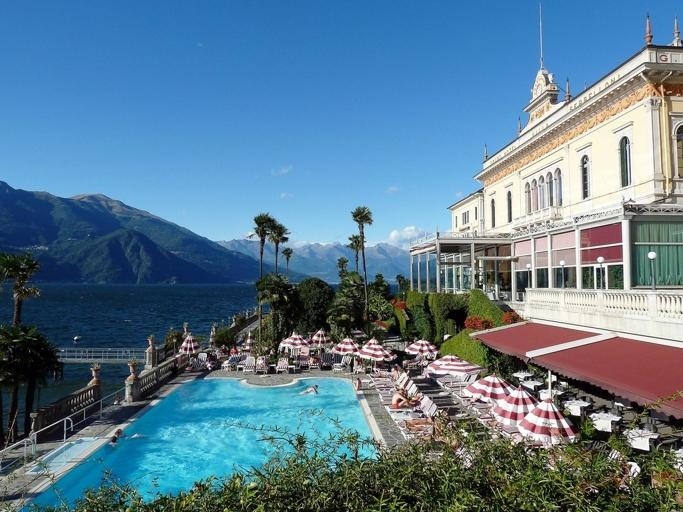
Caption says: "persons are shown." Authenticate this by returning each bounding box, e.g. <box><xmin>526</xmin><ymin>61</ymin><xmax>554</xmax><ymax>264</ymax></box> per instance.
<box><xmin>404</xmin><ymin>410</ymin><xmax>463</xmax><ymax>452</ymax></box>
<box><xmin>114</xmin><ymin>428</ymin><xmax>138</xmax><ymax>439</ymax></box>
<box><xmin>298</xmin><ymin>384</ymin><xmax>318</xmax><ymax>394</ymax></box>
<box><xmin>108</xmin><ymin>436</ymin><xmax>119</xmax><ymax>447</ymax></box>
<box><xmin>400</xmin><ymin>391</ymin><xmax>423</xmax><ymax>404</ymax></box>
<box><xmin>391</xmin><ymin>388</ymin><xmax>417</xmax><ymax>410</ymax></box>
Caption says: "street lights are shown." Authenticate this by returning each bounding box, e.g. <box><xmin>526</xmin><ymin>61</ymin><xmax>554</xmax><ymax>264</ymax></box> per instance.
<box><xmin>648</xmin><ymin>252</ymin><xmax>657</xmax><ymax>290</ymax></box>
<box><xmin>526</xmin><ymin>263</ymin><xmax>531</xmax><ymax>288</ymax></box>
<box><xmin>560</xmin><ymin>260</ymin><xmax>565</xmax><ymax>288</ymax></box>
<box><xmin>597</xmin><ymin>257</ymin><xmax>604</xmax><ymax>289</ymax></box>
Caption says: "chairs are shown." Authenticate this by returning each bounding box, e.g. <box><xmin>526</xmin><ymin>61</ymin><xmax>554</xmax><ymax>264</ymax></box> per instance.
<box><xmin>192</xmin><ymin>345</ymin><xmax>683</xmax><ymax>489</ymax></box>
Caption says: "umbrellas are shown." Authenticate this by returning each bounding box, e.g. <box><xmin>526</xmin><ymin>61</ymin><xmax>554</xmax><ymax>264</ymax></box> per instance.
<box><xmin>434</xmin><ymin>357</ymin><xmax>489</xmax><ymax>414</ymax></box>
<box><xmin>425</xmin><ymin>351</ymin><xmax>462</xmax><ymax>391</ymax></box>
<box><xmin>490</xmin><ymin>384</ymin><xmax>541</xmax><ymax>427</ymax></box>
<box><xmin>177</xmin><ymin>326</ymin><xmax>439</xmax><ymax>378</ymax></box>
<box><xmin>515</xmin><ymin>395</ymin><xmax>581</xmax><ymax>447</ymax></box>
<box><xmin>460</xmin><ymin>371</ymin><xmax>518</xmax><ymax>418</ymax></box>
